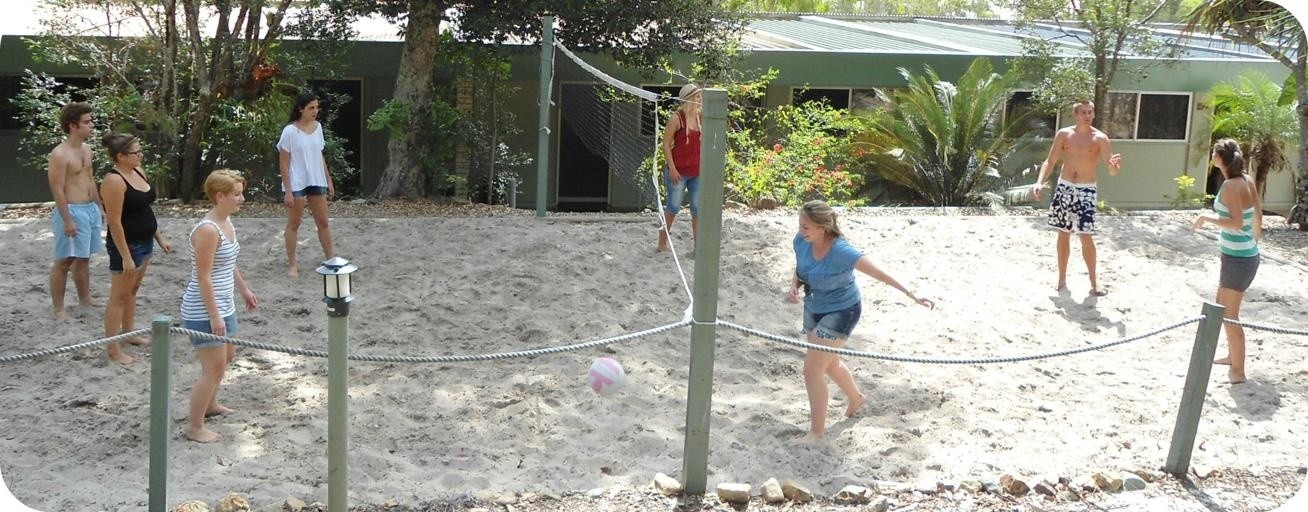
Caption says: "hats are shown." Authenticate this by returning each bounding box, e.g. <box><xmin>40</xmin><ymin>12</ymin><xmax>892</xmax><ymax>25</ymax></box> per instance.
<box><xmin>679</xmin><ymin>83</ymin><xmax>702</xmax><ymax>104</ymax></box>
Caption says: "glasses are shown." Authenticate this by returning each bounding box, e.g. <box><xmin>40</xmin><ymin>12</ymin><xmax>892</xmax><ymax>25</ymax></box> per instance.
<box><xmin>126</xmin><ymin>149</ymin><xmax>145</xmax><ymax>157</ymax></box>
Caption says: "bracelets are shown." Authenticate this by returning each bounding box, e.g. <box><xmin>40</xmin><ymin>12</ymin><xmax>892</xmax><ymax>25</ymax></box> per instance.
<box><xmin>906</xmin><ymin>289</ymin><xmax>917</xmax><ymax>295</ymax></box>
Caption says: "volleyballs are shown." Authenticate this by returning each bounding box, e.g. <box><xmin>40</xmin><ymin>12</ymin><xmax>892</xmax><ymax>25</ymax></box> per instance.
<box><xmin>586</xmin><ymin>356</ymin><xmax>627</xmax><ymax>398</ymax></box>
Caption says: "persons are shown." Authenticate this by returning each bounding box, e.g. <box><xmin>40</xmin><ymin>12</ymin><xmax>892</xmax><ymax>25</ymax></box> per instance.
<box><xmin>1034</xmin><ymin>100</ymin><xmax>1121</xmax><ymax>296</ymax></box>
<box><xmin>48</xmin><ymin>102</ymin><xmax>172</xmax><ymax>364</ymax></box>
<box><xmin>1190</xmin><ymin>139</ymin><xmax>1262</xmax><ymax>385</ymax></box>
<box><xmin>276</xmin><ymin>94</ymin><xmax>335</xmax><ymax>278</ymax></box>
<box><xmin>181</xmin><ymin>168</ymin><xmax>258</xmax><ymax>443</ymax></box>
<box><xmin>790</xmin><ymin>200</ymin><xmax>935</xmax><ymax>444</ymax></box>
<box><xmin>659</xmin><ymin>83</ymin><xmax>702</xmax><ymax>251</ymax></box>
